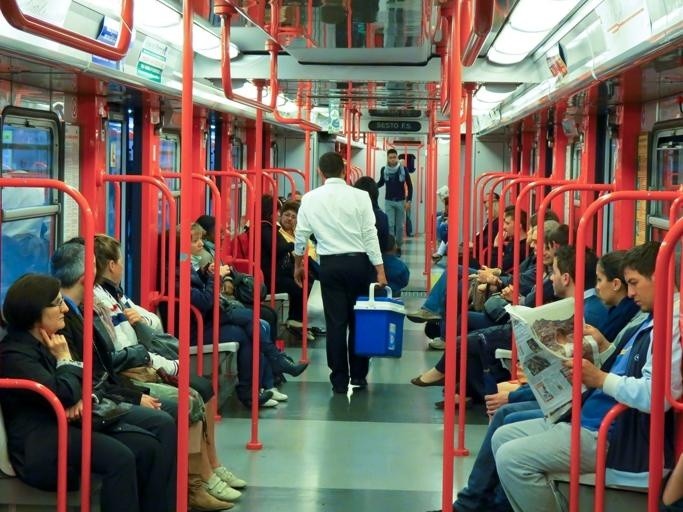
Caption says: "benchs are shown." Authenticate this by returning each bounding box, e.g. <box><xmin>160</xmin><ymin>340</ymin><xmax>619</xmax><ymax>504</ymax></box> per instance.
<box><xmin>494</xmin><ymin>349</ymin><xmax>682</xmax><ymax>511</ymax></box>
<box><xmin>0</xmin><ymin>294</ymin><xmax>290</xmax><ymax>511</ymax></box>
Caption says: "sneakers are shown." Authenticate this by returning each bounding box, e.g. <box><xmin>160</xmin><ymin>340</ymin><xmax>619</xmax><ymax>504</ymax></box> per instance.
<box><xmin>406</xmin><ymin>309</ymin><xmax>442</xmax><ymax>324</ymax></box>
<box><xmin>331</xmin><ymin>387</ymin><xmax>347</xmax><ymax>394</ymax></box>
<box><xmin>396</xmin><ymin>248</ymin><xmax>403</xmax><ymax>258</ymax></box>
<box><xmin>286</xmin><ymin>318</ymin><xmax>327</xmax><ymax>341</ymax></box>
<box><xmin>410</xmin><ymin>375</ymin><xmax>446</xmax><ymax>386</ymax></box>
<box><xmin>428</xmin><ymin>337</ymin><xmax>445</xmax><ymax>350</ymax></box>
<box><xmin>350</xmin><ymin>378</ymin><xmax>368</xmax><ymax>386</ymax></box>
<box><xmin>434</xmin><ymin>399</ymin><xmax>475</xmax><ymax>410</ymax></box>
<box><xmin>264</xmin><ymin>387</ymin><xmax>288</xmax><ymax>401</ymax></box>
<box><xmin>259</xmin><ymin>397</ymin><xmax>278</xmax><ymax>407</ymax></box>
<box><xmin>205</xmin><ymin>474</ymin><xmax>242</xmax><ymax>501</ymax></box>
<box><xmin>215</xmin><ymin>467</ymin><xmax>246</xmax><ymax>488</ymax></box>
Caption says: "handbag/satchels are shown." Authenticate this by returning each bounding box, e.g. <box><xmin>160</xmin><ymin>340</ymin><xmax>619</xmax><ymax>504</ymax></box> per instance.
<box><xmin>90</xmin><ymin>369</ymin><xmax>134</xmax><ymax>431</ymax></box>
<box><xmin>134</xmin><ymin>322</ymin><xmax>182</xmax><ymax>360</ymax></box>
<box><xmin>470</xmin><ymin>277</ymin><xmax>501</xmax><ymax>311</ymax></box>
<box><xmin>231</xmin><ymin>268</ymin><xmax>267</xmax><ymax>305</ymax></box>
<box><xmin>483</xmin><ymin>292</ymin><xmax>511</xmax><ymax>324</ymax></box>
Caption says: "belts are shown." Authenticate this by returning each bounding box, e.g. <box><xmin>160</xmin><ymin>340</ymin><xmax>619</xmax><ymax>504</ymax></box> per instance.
<box><xmin>330</xmin><ymin>252</ymin><xmax>368</xmax><ymax>258</ymax></box>
<box><xmin>384</xmin><ymin>197</ymin><xmax>405</xmax><ymax>202</ymax></box>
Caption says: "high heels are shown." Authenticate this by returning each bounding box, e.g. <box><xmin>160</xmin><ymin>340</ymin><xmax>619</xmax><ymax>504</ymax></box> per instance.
<box><xmin>236</xmin><ymin>386</ymin><xmax>273</xmax><ymax>408</ymax></box>
<box><xmin>270</xmin><ymin>348</ymin><xmax>308</xmax><ymax>383</ymax></box>
<box><xmin>431</xmin><ymin>252</ymin><xmax>442</xmax><ymax>265</ymax></box>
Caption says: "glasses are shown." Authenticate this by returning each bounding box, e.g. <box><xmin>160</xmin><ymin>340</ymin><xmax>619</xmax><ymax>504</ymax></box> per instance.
<box><xmin>46</xmin><ymin>299</ymin><xmax>63</xmax><ymax>308</ymax></box>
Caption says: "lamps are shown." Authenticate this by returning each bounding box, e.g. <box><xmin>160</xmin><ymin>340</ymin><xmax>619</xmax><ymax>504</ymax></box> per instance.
<box><xmin>469</xmin><ymin>83</ymin><xmax>520</xmax><ymax>117</ymax></box>
<box><xmin>69</xmin><ymin>0</ymin><xmax>241</xmax><ymax>62</ymax></box>
<box><xmin>484</xmin><ymin>1</ymin><xmax>586</xmax><ymax>64</ymax></box>
<box><xmin>213</xmin><ymin>78</ymin><xmax>297</xmax><ymax>113</ymax></box>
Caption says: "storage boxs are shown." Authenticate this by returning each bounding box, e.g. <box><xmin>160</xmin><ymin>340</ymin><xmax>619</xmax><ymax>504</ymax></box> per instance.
<box><xmin>352</xmin><ymin>282</ymin><xmax>408</xmax><ymax>358</ymax></box>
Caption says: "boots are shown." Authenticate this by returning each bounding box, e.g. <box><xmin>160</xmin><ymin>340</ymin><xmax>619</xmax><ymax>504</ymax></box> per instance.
<box><xmin>187</xmin><ymin>452</ymin><xmax>234</xmax><ymax>512</ymax></box>
